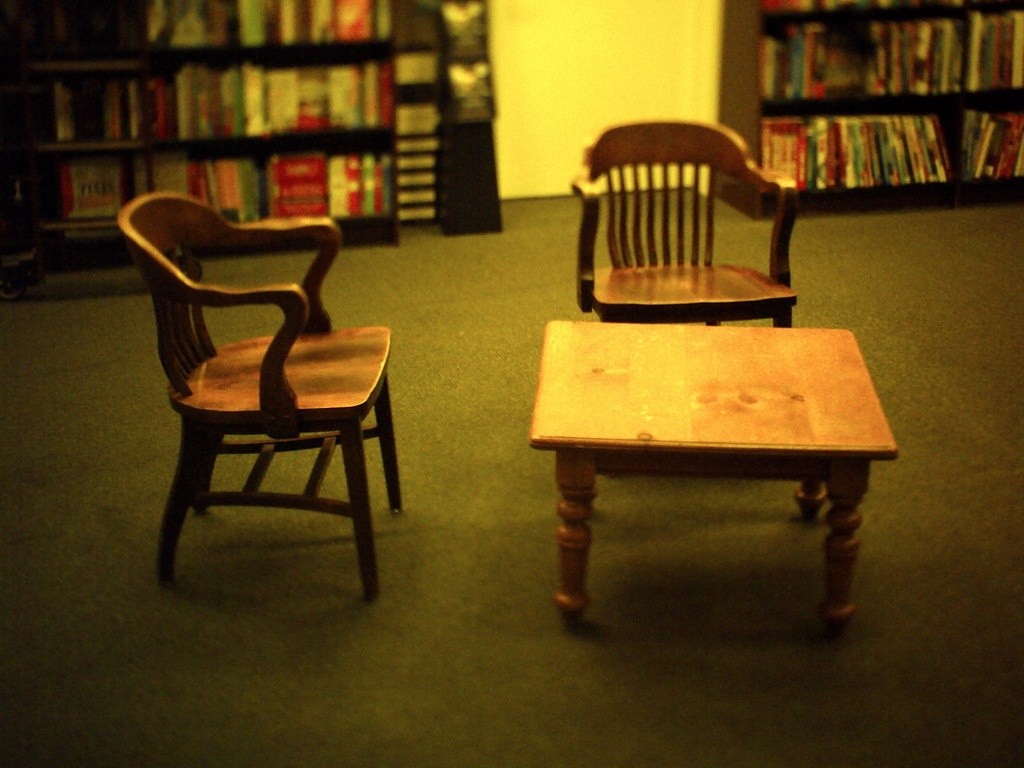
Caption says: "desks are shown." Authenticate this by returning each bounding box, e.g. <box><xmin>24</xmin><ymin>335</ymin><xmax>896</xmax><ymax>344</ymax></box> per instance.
<box><xmin>528</xmin><ymin>319</ymin><xmax>900</xmax><ymax>638</ymax></box>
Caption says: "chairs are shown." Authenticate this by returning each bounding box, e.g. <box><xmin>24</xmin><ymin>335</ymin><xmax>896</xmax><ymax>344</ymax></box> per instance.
<box><xmin>572</xmin><ymin>117</ymin><xmax>801</xmax><ymax>325</ymax></box>
<box><xmin>118</xmin><ymin>191</ymin><xmax>400</xmax><ymax>604</ymax></box>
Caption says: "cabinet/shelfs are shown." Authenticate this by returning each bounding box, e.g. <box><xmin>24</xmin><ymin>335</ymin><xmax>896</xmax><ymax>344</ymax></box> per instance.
<box><xmin>709</xmin><ymin>1</ymin><xmax>1023</xmax><ymax>218</ymax></box>
<box><xmin>0</xmin><ymin>2</ymin><xmax>401</xmax><ymax>274</ymax></box>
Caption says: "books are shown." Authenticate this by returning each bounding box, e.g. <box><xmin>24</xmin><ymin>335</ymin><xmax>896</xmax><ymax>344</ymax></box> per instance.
<box><xmin>43</xmin><ymin>0</ymin><xmax>395</xmax><ymax>221</ymax></box>
<box><xmin>759</xmin><ymin>0</ymin><xmax>1024</xmax><ymax>192</ymax></box>
